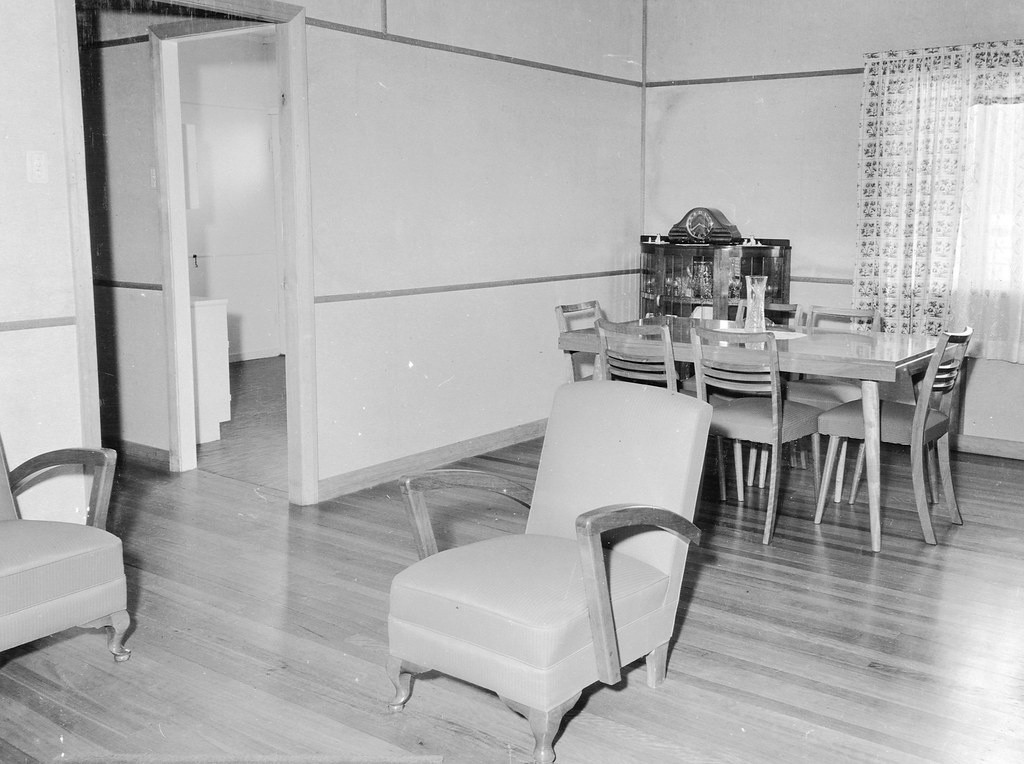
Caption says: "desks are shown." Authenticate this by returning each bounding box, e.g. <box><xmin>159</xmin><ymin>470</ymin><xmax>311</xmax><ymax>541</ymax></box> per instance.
<box><xmin>556</xmin><ymin>317</ymin><xmax>956</xmax><ymax>554</ymax></box>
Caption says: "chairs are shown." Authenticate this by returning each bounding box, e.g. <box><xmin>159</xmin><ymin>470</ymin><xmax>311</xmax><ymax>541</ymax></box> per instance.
<box><xmin>551</xmin><ymin>300</ymin><xmax>885</xmax><ymax>548</ymax></box>
<box><xmin>0</xmin><ymin>429</ymin><xmax>130</xmax><ymax>660</ymax></box>
<box><xmin>813</xmin><ymin>326</ymin><xmax>977</xmax><ymax>546</ymax></box>
<box><xmin>385</xmin><ymin>378</ymin><xmax>713</xmax><ymax>764</ymax></box>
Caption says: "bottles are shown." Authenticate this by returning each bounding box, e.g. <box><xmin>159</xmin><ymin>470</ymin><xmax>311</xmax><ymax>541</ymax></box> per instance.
<box><xmin>742</xmin><ymin>274</ymin><xmax>767</xmax><ymax>349</ymax></box>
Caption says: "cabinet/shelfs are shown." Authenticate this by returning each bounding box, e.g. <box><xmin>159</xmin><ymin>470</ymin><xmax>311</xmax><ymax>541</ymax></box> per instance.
<box><xmin>191</xmin><ymin>296</ymin><xmax>231</xmax><ymax>446</ymax></box>
